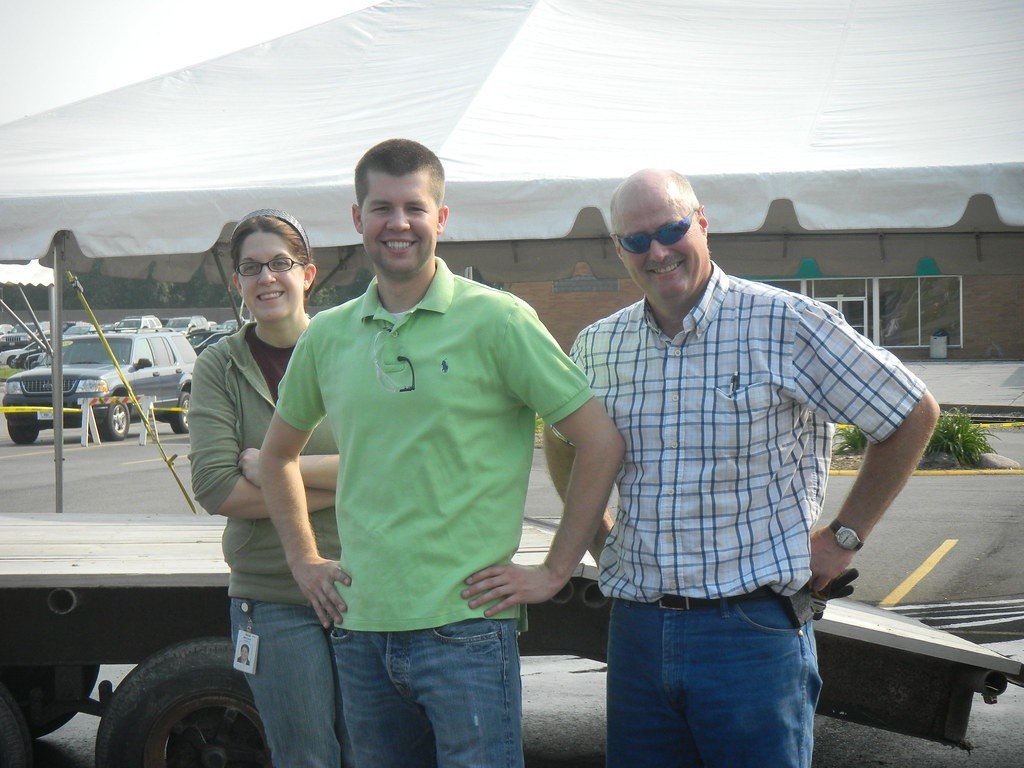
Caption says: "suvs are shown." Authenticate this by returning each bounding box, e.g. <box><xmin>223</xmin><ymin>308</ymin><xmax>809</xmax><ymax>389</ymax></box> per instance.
<box><xmin>0</xmin><ymin>313</ymin><xmax>311</xmax><ymax>371</ymax></box>
<box><xmin>2</xmin><ymin>332</ymin><xmax>198</xmax><ymax>445</ymax></box>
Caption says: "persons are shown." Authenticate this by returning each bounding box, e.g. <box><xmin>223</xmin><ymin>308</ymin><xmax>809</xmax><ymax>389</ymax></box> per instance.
<box><xmin>542</xmin><ymin>165</ymin><xmax>942</xmax><ymax>768</ymax></box>
<box><xmin>185</xmin><ymin>207</ymin><xmax>349</xmax><ymax>768</ymax></box>
<box><xmin>258</xmin><ymin>136</ymin><xmax>624</xmax><ymax>768</ymax></box>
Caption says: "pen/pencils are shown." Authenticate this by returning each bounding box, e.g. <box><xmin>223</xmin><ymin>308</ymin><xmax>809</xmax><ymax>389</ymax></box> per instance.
<box><xmin>729</xmin><ymin>370</ymin><xmax>739</xmax><ymax>394</ymax></box>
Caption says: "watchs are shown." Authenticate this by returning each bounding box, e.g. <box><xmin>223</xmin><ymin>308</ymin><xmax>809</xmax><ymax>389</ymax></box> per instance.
<box><xmin>829</xmin><ymin>518</ymin><xmax>864</xmax><ymax>552</ymax></box>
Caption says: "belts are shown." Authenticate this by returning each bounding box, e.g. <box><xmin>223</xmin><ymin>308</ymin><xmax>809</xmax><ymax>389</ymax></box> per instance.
<box><xmin>645</xmin><ymin>585</ymin><xmax>776</xmax><ymax>611</ymax></box>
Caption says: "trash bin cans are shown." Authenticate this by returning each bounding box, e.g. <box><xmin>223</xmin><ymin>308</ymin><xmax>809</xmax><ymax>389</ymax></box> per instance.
<box><xmin>929</xmin><ymin>334</ymin><xmax>948</xmax><ymax>358</ymax></box>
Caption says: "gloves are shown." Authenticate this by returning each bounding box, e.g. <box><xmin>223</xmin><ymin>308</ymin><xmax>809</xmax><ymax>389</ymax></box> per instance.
<box><xmin>808</xmin><ymin>567</ymin><xmax>860</xmax><ymax>621</ymax></box>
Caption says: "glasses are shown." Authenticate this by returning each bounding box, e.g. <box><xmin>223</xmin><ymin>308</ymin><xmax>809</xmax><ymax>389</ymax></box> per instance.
<box><xmin>615</xmin><ymin>209</ymin><xmax>697</xmax><ymax>255</ymax></box>
<box><xmin>235</xmin><ymin>258</ymin><xmax>304</xmax><ymax>276</ymax></box>
